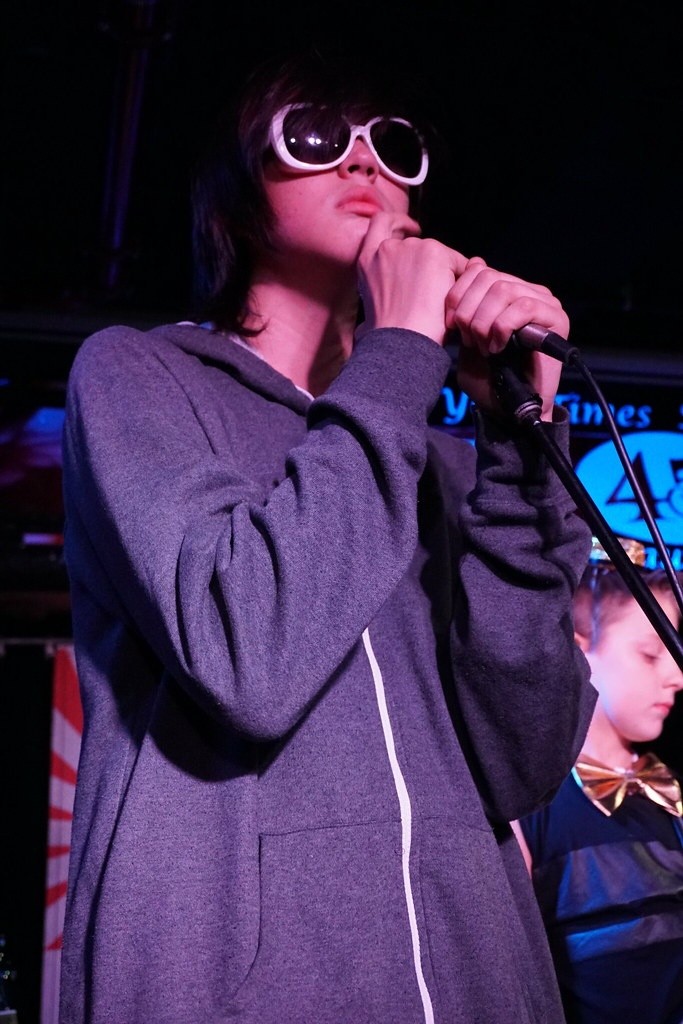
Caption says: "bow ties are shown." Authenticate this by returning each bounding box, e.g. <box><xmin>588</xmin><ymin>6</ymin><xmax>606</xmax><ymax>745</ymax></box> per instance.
<box><xmin>571</xmin><ymin>753</ymin><xmax>683</xmax><ymax>817</ymax></box>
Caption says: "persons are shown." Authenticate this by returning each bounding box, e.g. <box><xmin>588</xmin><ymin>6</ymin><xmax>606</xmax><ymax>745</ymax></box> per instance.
<box><xmin>60</xmin><ymin>62</ymin><xmax>682</xmax><ymax>1024</ymax></box>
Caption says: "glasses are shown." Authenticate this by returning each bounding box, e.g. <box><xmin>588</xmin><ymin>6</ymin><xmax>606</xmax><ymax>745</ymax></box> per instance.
<box><xmin>589</xmin><ymin>562</ymin><xmax>615</xmax><ymax>645</ymax></box>
<box><xmin>261</xmin><ymin>102</ymin><xmax>429</xmax><ymax>186</ymax></box>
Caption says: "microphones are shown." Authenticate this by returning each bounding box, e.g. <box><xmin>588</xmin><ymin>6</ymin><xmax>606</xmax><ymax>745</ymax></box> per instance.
<box><xmin>355</xmin><ymin>279</ymin><xmax>582</xmax><ymax>367</ymax></box>
